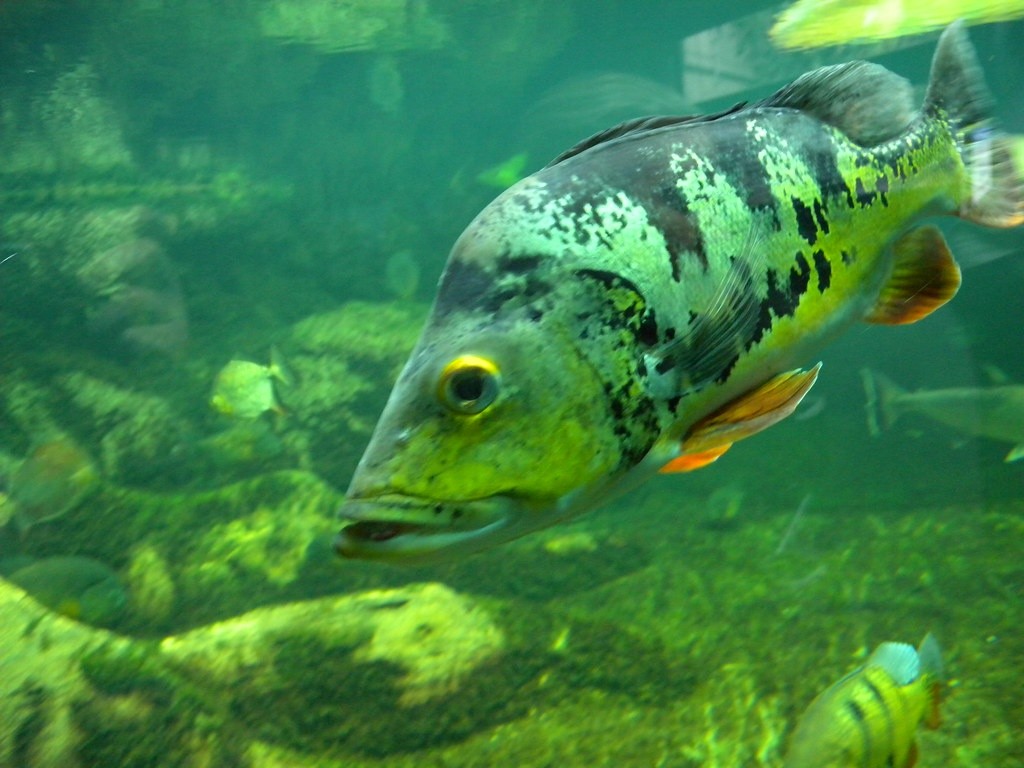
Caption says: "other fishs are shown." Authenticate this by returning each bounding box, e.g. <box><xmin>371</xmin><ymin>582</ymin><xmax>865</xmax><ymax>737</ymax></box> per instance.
<box><xmin>206</xmin><ymin>343</ymin><xmax>294</xmax><ymax>421</ymax></box>
<box><xmin>785</xmin><ymin>631</ymin><xmax>948</xmax><ymax>768</ymax></box>
<box><xmin>327</xmin><ymin>20</ymin><xmax>1024</xmax><ymax>569</ymax></box>
